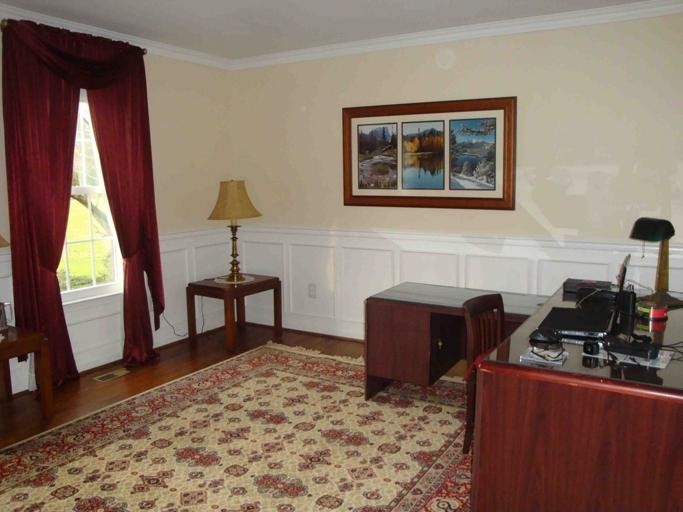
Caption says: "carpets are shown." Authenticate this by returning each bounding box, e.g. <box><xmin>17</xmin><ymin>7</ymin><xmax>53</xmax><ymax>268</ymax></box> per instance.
<box><xmin>1</xmin><ymin>340</ymin><xmax>471</xmax><ymax>511</ymax></box>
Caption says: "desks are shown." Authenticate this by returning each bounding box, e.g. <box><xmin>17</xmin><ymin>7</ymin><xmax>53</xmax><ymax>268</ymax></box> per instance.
<box><xmin>471</xmin><ymin>278</ymin><xmax>682</xmax><ymax>512</ymax></box>
<box><xmin>186</xmin><ymin>273</ymin><xmax>281</xmax><ymax>352</ymax></box>
<box><xmin>364</xmin><ymin>279</ymin><xmax>551</xmax><ymax>400</ymax></box>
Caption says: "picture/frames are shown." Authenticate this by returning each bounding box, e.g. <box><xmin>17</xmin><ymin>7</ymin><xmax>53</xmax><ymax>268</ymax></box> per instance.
<box><xmin>341</xmin><ymin>96</ymin><xmax>516</xmax><ymax>212</ymax></box>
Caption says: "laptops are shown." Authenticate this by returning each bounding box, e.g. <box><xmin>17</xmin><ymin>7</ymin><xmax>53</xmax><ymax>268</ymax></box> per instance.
<box><xmin>536</xmin><ymin>253</ymin><xmax>632</xmax><ymax>338</ymax></box>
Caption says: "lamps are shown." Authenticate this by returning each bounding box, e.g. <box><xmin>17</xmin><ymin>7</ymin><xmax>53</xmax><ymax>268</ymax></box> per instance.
<box><xmin>207</xmin><ymin>179</ymin><xmax>260</xmax><ymax>284</ymax></box>
<box><xmin>630</xmin><ymin>217</ymin><xmax>682</xmax><ymax>312</ymax></box>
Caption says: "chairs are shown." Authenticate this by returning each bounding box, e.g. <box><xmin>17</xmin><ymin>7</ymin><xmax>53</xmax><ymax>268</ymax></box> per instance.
<box><xmin>464</xmin><ymin>294</ymin><xmax>509</xmax><ymax>453</ymax></box>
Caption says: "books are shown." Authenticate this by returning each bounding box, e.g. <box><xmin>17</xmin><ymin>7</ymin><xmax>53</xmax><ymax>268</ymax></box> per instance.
<box><xmin>563</xmin><ymin>277</ymin><xmax>612</xmax><ymax>294</ymax></box>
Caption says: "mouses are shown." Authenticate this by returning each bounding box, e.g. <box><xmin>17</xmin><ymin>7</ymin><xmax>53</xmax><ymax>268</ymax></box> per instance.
<box><xmin>529</xmin><ymin>328</ymin><xmax>559</xmax><ymax>344</ymax></box>
